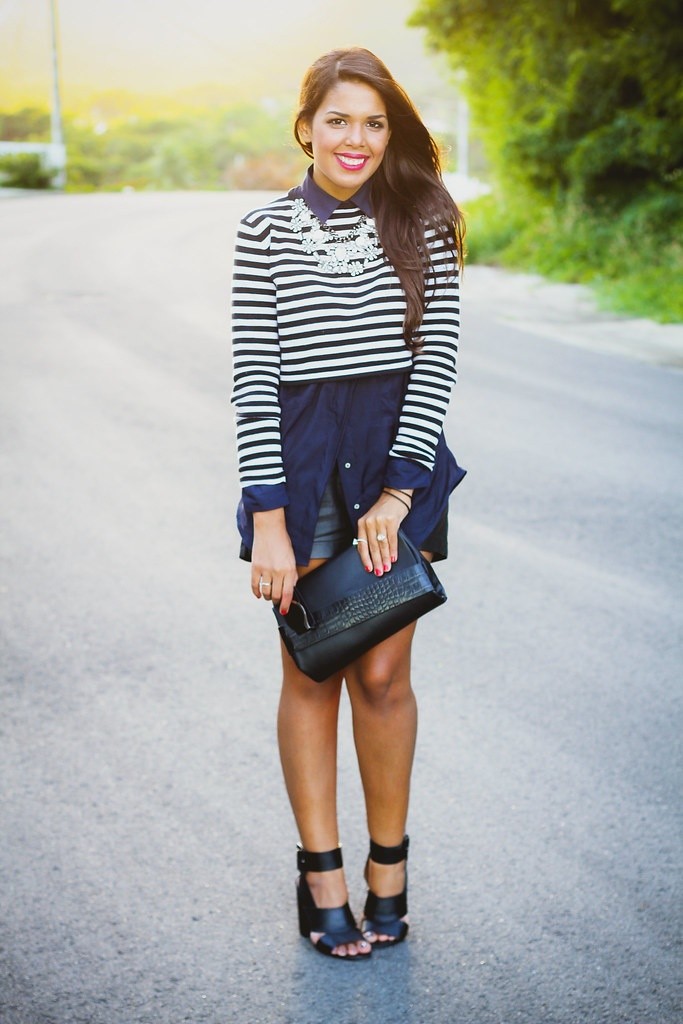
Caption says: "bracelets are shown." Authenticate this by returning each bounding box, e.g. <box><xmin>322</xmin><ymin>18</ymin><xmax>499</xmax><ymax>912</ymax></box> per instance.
<box><xmin>380</xmin><ymin>489</ymin><xmax>411</xmax><ymax>515</ymax></box>
<box><xmin>392</xmin><ymin>485</ymin><xmax>414</xmax><ymax>501</ymax></box>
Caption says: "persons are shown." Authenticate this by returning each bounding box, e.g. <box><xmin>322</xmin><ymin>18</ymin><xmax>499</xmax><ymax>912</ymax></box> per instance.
<box><xmin>231</xmin><ymin>47</ymin><xmax>469</xmax><ymax>965</ymax></box>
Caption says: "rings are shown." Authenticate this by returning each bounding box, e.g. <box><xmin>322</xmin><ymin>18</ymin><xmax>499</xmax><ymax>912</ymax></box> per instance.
<box><xmin>262</xmin><ymin>581</ymin><xmax>272</xmax><ymax>587</ymax></box>
<box><xmin>376</xmin><ymin>533</ymin><xmax>387</xmax><ymax>542</ymax></box>
<box><xmin>351</xmin><ymin>537</ymin><xmax>369</xmax><ymax>546</ymax></box>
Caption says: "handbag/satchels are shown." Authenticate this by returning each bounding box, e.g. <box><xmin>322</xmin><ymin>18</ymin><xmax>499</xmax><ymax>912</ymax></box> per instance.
<box><xmin>271</xmin><ymin>526</ymin><xmax>445</xmax><ymax>684</ymax></box>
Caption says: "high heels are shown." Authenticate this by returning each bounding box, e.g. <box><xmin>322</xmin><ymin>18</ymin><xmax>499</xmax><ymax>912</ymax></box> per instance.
<box><xmin>294</xmin><ymin>839</ymin><xmax>372</xmax><ymax>961</ymax></box>
<box><xmin>359</xmin><ymin>836</ymin><xmax>410</xmax><ymax>950</ymax></box>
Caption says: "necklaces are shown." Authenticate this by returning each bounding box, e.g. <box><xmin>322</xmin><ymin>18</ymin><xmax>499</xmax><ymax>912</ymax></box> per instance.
<box><xmin>292</xmin><ymin>193</ymin><xmax>384</xmax><ymax>277</ymax></box>
